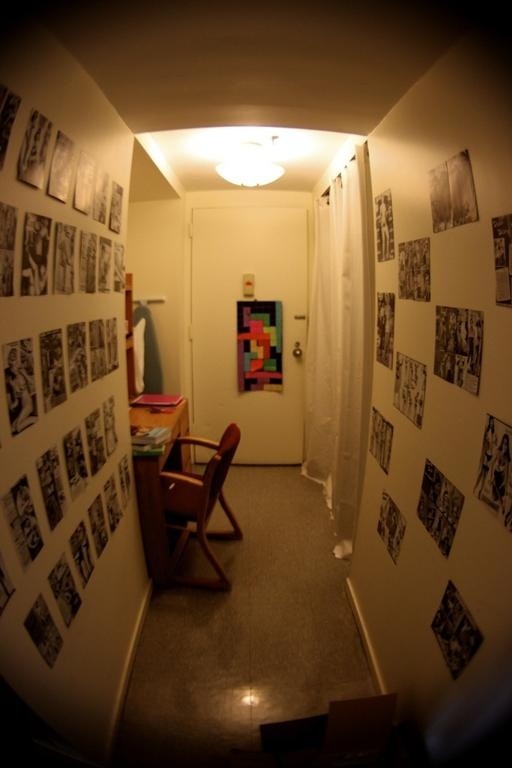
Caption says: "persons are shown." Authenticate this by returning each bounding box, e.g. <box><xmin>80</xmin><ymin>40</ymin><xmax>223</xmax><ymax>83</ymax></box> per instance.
<box><xmin>376</xmin><ymin>196</ymin><xmax>393</xmax><ymax>260</ymax></box>
<box><xmin>6</xmin><ymin>348</ymin><xmax>37</xmax><ymax>432</ymax></box>
<box><xmin>473</xmin><ymin>415</ymin><xmax>511</xmax><ymax>516</ymax></box>
<box><xmin>435</xmin><ymin>310</ymin><xmax>482</xmax><ymax>389</ymax></box>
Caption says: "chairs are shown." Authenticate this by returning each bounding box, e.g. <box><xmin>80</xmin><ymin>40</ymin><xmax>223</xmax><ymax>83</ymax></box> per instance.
<box><xmin>159</xmin><ymin>424</ymin><xmax>244</xmax><ymax>593</ymax></box>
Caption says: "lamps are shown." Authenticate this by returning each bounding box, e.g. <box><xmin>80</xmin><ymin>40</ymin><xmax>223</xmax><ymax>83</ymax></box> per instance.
<box><xmin>215</xmin><ymin>143</ymin><xmax>285</xmax><ymax>187</ymax></box>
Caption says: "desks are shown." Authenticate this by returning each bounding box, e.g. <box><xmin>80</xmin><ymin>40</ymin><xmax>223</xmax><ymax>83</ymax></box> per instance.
<box><xmin>129</xmin><ymin>394</ymin><xmax>191</xmax><ymax>587</ymax></box>
